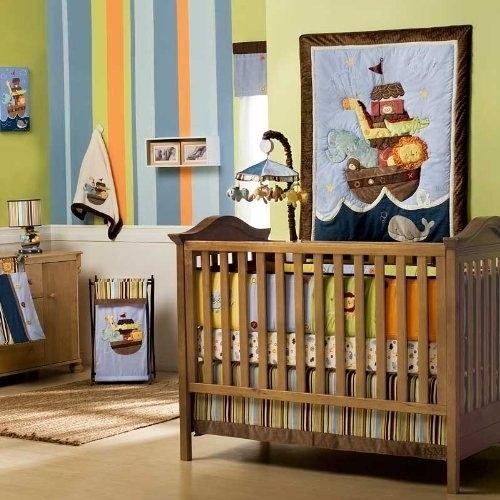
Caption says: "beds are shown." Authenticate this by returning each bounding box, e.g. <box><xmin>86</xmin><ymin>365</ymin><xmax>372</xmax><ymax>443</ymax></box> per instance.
<box><xmin>168</xmin><ymin>215</ymin><xmax>500</xmax><ymax>495</ymax></box>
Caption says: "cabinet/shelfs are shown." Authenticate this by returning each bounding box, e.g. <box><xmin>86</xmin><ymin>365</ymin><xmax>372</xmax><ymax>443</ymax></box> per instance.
<box><xmin>0</xmin><ymin>249</ymin><xmax>83</xmax><ymax>378</ymax></box>
<box><xmin>145</xmin><ymin>136</ymin><xmax>220</xmax><ymax>167</ymax></box>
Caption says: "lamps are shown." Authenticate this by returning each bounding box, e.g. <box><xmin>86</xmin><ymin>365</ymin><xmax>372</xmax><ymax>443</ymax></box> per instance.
<box><xmin>7</xmin><ymin>199</ymin><xmax>43</xmax><ymax>253</ymax></box>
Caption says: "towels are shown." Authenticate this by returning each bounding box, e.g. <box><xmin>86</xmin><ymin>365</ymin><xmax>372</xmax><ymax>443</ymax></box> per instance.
<box><xmin>70</xmin><ymin>124</ymin><xmax>125</xmax><ymax>239</ymax></box>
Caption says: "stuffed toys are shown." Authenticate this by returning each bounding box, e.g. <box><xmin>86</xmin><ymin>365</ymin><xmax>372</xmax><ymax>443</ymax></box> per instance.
<box><xmin>225</xmin><ymin>184</ymin><xmax>309</xmax><ymax>207</ymax></box>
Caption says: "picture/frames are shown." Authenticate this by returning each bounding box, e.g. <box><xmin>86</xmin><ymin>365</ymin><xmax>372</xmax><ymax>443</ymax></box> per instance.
<box><xmin>181</xmin><ymin>140</ymin><xmax>207</xmax><ymax>164</ymax></box>
<box><xmin>150</xmin><ymin>141</ymin><xmax>180</xmax><ymax>165</ymax></box>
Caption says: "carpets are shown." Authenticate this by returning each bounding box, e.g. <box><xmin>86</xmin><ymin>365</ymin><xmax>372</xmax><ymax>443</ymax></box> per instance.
<box><xmin>0</xmin><ymin>373</ymin><xmax>179</xmax><ymax>445</ymax></box>
